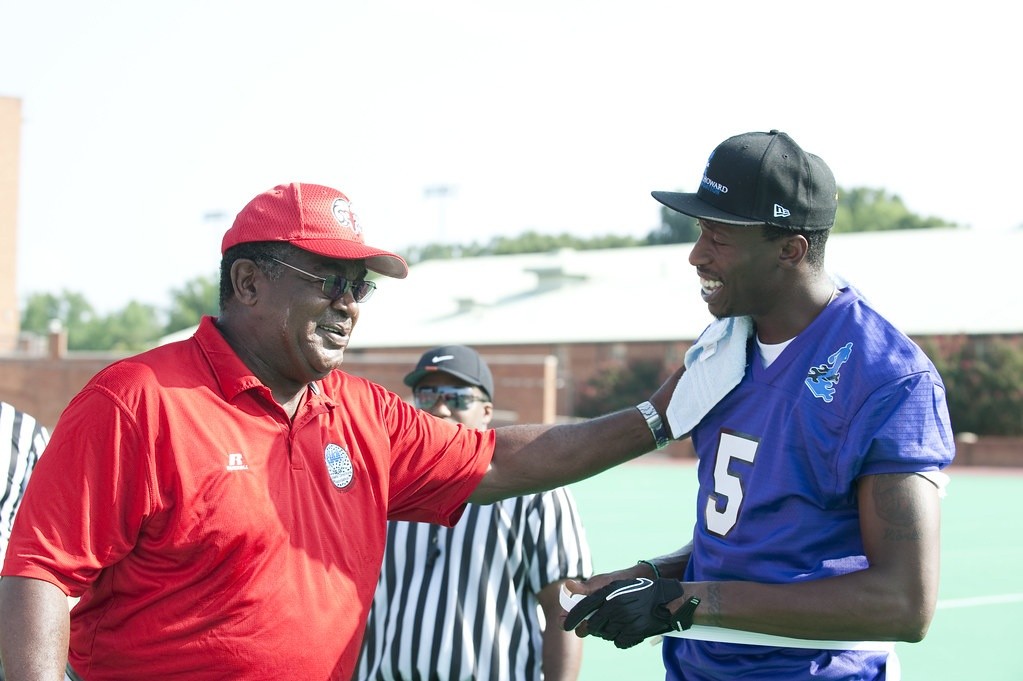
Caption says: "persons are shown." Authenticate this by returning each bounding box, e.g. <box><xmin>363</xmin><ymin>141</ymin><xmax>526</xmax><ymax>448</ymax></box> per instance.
<box><xmin>348</xmin><ymin>345</ymin><xmax>594</xmax><ymax>681</ymax></box>
<box><xmin>0</xmin><ymin>398</ymin><xmax>82</xmax><ymax>614</ymax></box>
<box><xmin>560</xmin><ymin>129</ymin><xmax>957</xmax><ymax>681</ymax></box>
<box><xmin>1</xmin><ymin>180</ymin><xmax>714</xmax><ymax>681</ymax></box>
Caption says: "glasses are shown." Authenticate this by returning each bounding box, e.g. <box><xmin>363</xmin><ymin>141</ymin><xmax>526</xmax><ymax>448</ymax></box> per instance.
<box><xmin>415</xmin><ymin>384</ymin><xmax>489</xmax><ymax>410</ymax></box>
<box><xmin>253</xmin><ymin>250</ymin><xmax>376</xmax><ymax>303</ymax></box>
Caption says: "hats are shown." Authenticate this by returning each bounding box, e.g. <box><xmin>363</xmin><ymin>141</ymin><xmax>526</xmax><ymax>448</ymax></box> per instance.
<box><xmin>652</xmin><ymin>130</ymin><xmax>838</xmax><ymax>231</ymax></box>
<box><xmin>403</xmin><ymin>344</ymin><xmax>495</xmax><ymax>402</ymax></box>
<box><xmin>220</xmin><ymin>183</ymin><xmax>408</xmax><ymax>279</ymax></box>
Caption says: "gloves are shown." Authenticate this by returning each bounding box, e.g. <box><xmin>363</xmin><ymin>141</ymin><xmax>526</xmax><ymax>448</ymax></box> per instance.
<box><xmin>564</xmin><ymin>569</ymin><xmax>699</xmax><ymax>648</ymax></box>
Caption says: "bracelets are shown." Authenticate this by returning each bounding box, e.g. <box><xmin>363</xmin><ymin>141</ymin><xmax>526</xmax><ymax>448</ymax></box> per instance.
<box><xmin>637</xmin><ymin>559</ymin><xmax>662</xmax><ymax>581</ymax></box>
<box><xmin>634</xmin><ymin>400</ymin><xmax>669</xmax><ymax>451</ymax></box>
